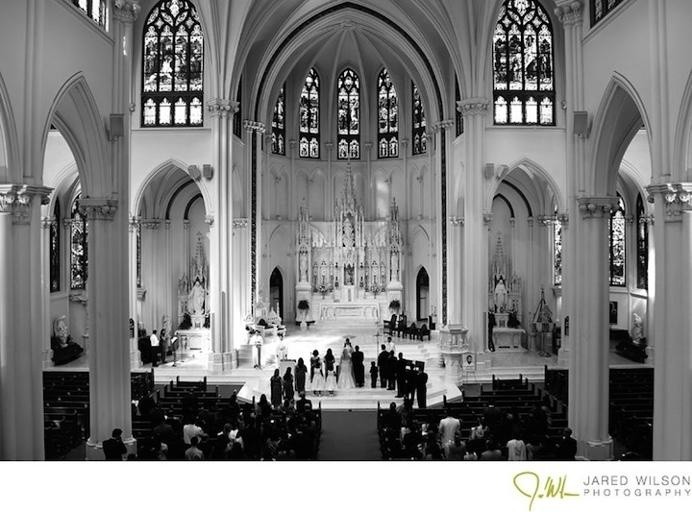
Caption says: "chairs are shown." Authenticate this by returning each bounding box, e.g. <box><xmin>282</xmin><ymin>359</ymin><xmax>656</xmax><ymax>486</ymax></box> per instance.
<box><xmin>382</xmin><ymin>313</ymin><xmax>431</xmax><ymax>342</ymax></box>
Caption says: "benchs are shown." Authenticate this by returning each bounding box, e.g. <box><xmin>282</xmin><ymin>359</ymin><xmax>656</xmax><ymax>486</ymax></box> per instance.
<box><xmin>376</xmin><ymin>363</ymin><xmax>654</xmax><ymax>461</ymax></box>
<box><xmin>42</xmin><ymin>367</ymin><xmax>324</xmax><ymax>461</ymax></box>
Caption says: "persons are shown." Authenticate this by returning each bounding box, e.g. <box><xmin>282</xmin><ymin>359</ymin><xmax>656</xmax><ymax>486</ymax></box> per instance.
<box><xmin>187</xmin><ymin>280</ymin><xmax>204</xmax><ymax>315</ymax></box>
<box><xmin>493</xmin><ymin>279</ymin><xmax>508</xmax><ymax>313</ymax></box>
<box><xmin>101</xmin><ymin>325</ymin><xmax>577</xmax><ymax>460</ymax></box>
<box><xmin>54</xmin><ymin>316</ymin><xmax>79</xmax><ymax>362</ymax></box>
<box><xmin>630</xmin><ymin>312</ymin><xmax>642</xmax><ymax>344</ymax></box>
